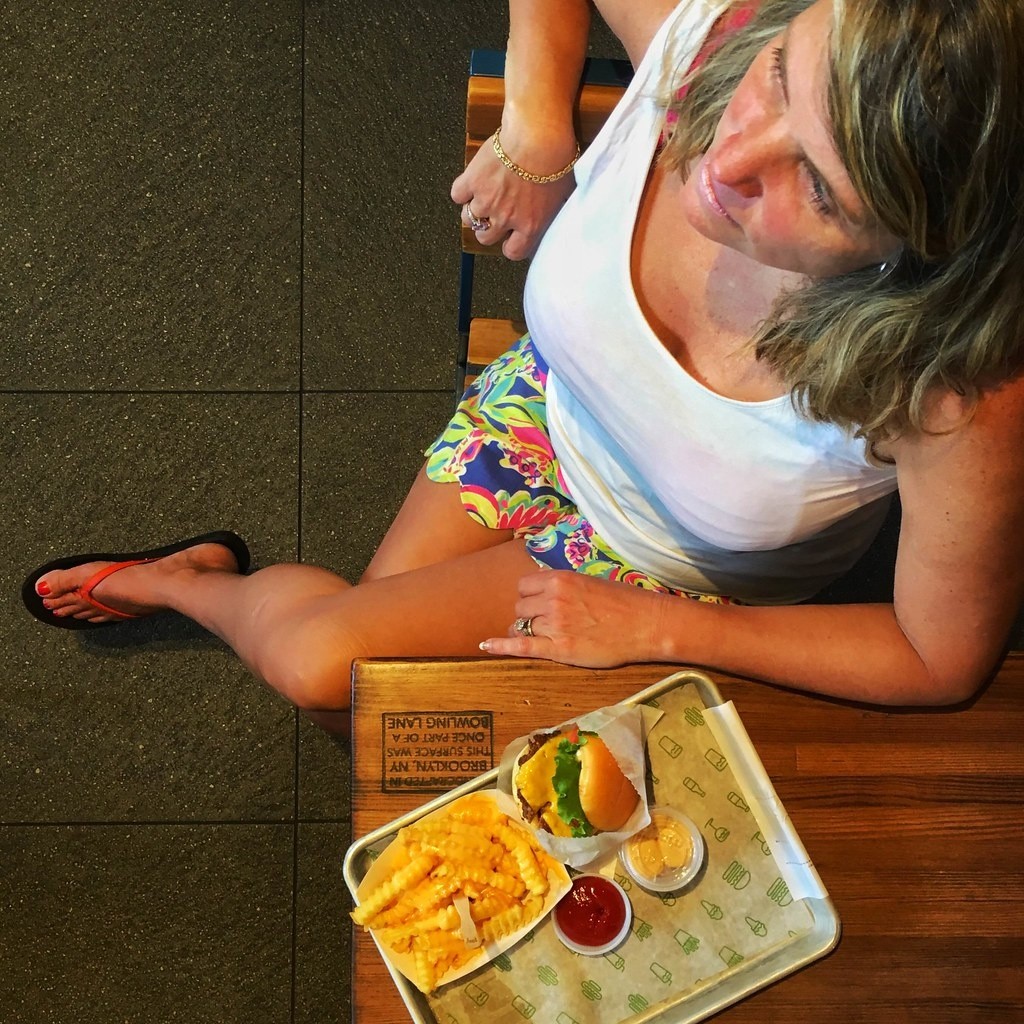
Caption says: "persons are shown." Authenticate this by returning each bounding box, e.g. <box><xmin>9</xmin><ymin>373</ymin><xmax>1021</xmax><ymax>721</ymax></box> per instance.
<box><xmin>20</xmin><ymin>0</ymin><xmax>1024</xmax><ymax>735</ymax></box>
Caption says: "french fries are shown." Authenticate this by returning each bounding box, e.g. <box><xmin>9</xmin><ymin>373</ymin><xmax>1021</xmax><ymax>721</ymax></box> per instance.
<box><xmin>349</xmin><ymin>807</ymin><xmax>550</xmax><ymax>992</ymax></box>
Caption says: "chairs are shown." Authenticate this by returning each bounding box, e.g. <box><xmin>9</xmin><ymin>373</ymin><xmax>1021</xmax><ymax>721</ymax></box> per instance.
<box><xmin>455</xmin><ymin>47</ymin><xmax>635</xmax><ymax>418</ymax></box>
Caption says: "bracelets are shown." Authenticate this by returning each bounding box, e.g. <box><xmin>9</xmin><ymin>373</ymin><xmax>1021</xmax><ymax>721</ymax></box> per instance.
<box><xmin>493</xmin><ymin>127</ymin><xmax>581</xmax><ymax>184</ymax></box>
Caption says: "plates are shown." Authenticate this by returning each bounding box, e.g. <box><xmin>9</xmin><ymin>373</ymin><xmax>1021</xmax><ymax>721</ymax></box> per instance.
<box><xmin>355</xmin><ymin>789</ymin><xmax>573</xmax><ymax>992</ymax></box>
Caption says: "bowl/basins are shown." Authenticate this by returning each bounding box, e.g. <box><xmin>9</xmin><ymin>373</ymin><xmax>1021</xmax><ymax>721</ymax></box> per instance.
<box><xmin>621</xmin><ymin>808</ymin><xmax>705</xmax><ymax>893</ymax></box>
<box><xmin>550</xmin><ymin>872</ymin><xmax>632</xmax><ymax>957</ymax></box>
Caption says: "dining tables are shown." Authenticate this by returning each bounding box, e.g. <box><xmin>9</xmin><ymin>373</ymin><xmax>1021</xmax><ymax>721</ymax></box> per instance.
<box><xmin>350</xmin><ymin>642</ymin><xmax>1024</xmax><ymax>1024</ymax></box>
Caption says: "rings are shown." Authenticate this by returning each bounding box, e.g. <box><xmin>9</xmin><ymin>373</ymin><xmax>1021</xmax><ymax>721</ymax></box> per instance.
<box><xmin>466</xmin><ymin>205</ymin><xmax>490</xmax><ymax>232</ymax></box>
<box><xmin>515</xmin><ymin>618</ymin><xmax>533</xmax><ymax>636</ymax></box>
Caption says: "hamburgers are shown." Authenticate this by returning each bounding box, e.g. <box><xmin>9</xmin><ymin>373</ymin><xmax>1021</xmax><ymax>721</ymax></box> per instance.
<box><xmin>512</xmin><ymin>721</ymin><xmax>639</xmax><ymax>839</ymax></box>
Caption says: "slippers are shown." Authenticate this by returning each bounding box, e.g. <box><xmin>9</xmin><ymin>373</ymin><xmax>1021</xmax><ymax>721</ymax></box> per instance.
<box><xmin>21</xmin><ymin>531</ymin><xmax>252</xmax><ymax>631</ymax></box>
